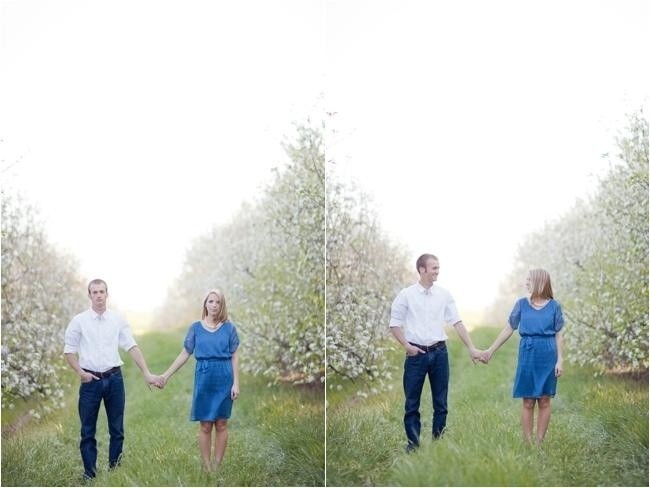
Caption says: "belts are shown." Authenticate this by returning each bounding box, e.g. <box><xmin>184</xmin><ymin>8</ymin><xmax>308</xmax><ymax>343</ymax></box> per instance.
<box><xmin>407</xmin><ymin>340</ymin><xmax>444</xmax><ymax>351</ymax></box>
<box><xmin>81</xmin><ymin>366</ymin><xmax>119</xmax><ymax>379</ymax></box>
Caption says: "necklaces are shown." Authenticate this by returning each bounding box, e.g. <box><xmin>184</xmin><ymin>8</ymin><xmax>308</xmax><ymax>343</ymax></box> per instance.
<box><xmin>533</xmin><ymin>298</ymin><xmax>547</xmax><ymax>308</ymax></box>
<box><xmin>204</xmin><ymin>315</ymin><xmax>221</xmax><ymax>329</ymax></box>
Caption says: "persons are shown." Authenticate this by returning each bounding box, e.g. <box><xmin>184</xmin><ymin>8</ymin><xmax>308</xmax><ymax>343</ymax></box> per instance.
<box><xmin>156</xmin><ymin>289</ymin><xmax>242</xmax><ymax>472</ymax></box>
<box><xmin>479</xmin><ymin>268</ymin><xmax>565</xmax><ymax>448</ymax></box>
<box><xmin>389</xmin><ymin>252</ymin><xmax>484</xmax><ymax>454</ymax></box>
<box><xmin>62</xmin><ymin>278</ymin><xmax>163</xmax><ymax>481</ymax></box>
